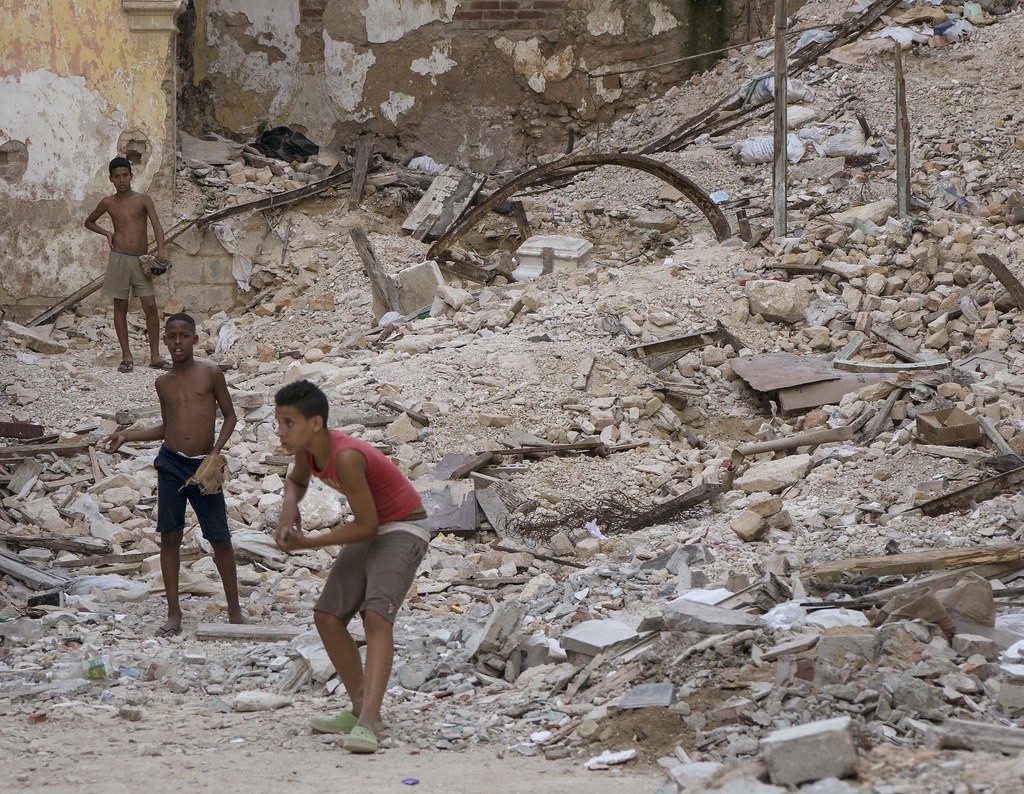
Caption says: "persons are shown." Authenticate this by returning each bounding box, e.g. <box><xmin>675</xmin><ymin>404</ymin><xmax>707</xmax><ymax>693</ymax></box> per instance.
<box><xmin>269</xmin><ymin>377</ymin><xmax>431</xmax><ymax>754</ymax></box>
<box><xmin>83</xmin><ymin>156</ymin><xmax>174</xmax><ymax>374</ymax></box>
<box><xmin>101</xmin><ymin>312</ymin><xmax>256</xmax><ymax>638</ymax></box>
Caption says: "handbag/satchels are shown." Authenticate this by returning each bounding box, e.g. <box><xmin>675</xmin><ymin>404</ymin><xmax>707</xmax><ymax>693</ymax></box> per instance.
<box><xmin>277</xmin><ymin>130</ymin><xmax>320</xmax><ymax>164</ymax></box>
<box><xmin>253</xmin><ymin>126</ymin><xmax>294</xmax><ymax>158</ymax></box>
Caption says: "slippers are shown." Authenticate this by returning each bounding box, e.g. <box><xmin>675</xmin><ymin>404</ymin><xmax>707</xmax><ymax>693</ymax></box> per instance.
<box><xmin>155</xmin><ymin>624</ymin><xmax>183</xmax><ymax>638</ymax></box>
<box><xmin>309</xmin><ymin>708</ymin><xmax>385</xmax><ymax>733</ymax></box>
<box><xmin>118</xmin><ymin>360</ymin><xmax>134</xmax><ymax>373</ymax></box>
<box><xmin>148</xmin><ymin>359</ymin><xmax>173</xmax><ymax>371</ymax></box>
<box><xmin>342</xmin><ymin>725</ymin><xmax>378</xmax><ymax>753</ymax></box>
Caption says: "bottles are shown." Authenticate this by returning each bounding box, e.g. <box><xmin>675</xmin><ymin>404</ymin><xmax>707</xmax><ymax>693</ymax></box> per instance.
<box><xmin>933</xmin><ymin>19</ymin><xmax>955</xmax><ymax>35</ymax></box>
<box><xmin>944</xmin><ymin>186</ymin><xmax>967</xmax><ymax>209</ymax></box>
<box><xmin>45</xmin><ymin>655</ymin><xmax>115</xmax><ymax>679</ymax></box>
